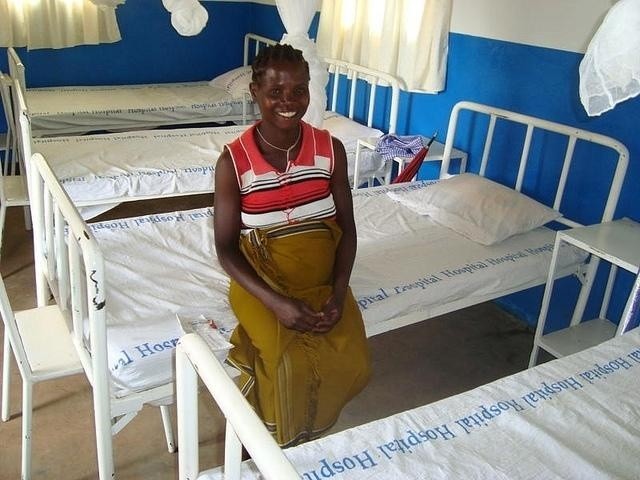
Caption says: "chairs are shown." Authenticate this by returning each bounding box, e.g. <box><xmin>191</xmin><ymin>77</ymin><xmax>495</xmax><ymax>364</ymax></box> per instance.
<box><xmin>0</xmin><ymin>266</ymin><xmax>176</xmax><ymax>480</ymax></box>
<box><xmin>0</xmin><ymin>149</ymin><xmax>30</xmax><ymax>250</ymax></box>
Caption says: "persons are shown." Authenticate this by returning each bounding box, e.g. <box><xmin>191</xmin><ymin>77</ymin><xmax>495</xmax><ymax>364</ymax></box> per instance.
<box><xmin>213</xmin><ymin>43</ymin><xmax>371</xmax><ymax>460</ymax></box>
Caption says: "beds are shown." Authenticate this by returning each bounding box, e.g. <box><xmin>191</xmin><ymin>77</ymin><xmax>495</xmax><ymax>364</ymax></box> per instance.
<box><xmin>177</xmin><ymin>324</ymin><xmax>640</xmax><ymax>480</ymax></box>
<box><xmin>4</xmin><ymin>28</ymin><xmax>282</xmax><ymax>134</ymax></box>
<box><xmin>24</xmin><ymin>100</ymin><xmax>631</xmax><ymax>480</ymax></box>
<box><xmin>13</xmin><ymin>57</ymin><xmax>401</xmax><ymax>205</ymax></box>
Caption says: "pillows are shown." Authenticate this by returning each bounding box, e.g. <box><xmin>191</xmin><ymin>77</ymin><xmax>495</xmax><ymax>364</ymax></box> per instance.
<box><xmin>386</xmin><ymin>172</ymin><xmax>562</xmax><ymax>249</ymax></box>
<box><xmin>205</xmin><ymin>67</ymin><xmax>256</xmax><ymax>102</ymax></box>
<box><xmin>316</xmin><ymin>108</ymin><xmax>380</xmax><ymax>150</ymax></box>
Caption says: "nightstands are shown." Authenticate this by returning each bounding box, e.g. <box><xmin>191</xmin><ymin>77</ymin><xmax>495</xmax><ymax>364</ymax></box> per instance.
<box><xmin>353</xmin><ymin>135</ymin><xmax>469</xmax><ymax>187</ymax></box>
<box><xmin>527</xmin><ymin>213</ymin><xmax>639</xmax><ymax>367</ymax></box>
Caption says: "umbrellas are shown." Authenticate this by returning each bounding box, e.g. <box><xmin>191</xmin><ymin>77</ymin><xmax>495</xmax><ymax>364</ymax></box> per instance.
<box><xmin>391</xmin><ymin>132</ymin><xmax>438</xmax><ymax>184</ymax></box>
